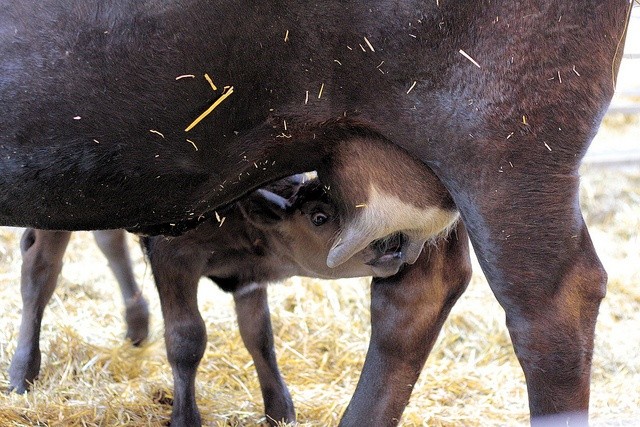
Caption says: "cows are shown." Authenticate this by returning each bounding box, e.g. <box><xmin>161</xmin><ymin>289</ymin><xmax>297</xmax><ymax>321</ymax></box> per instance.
<box><xmin>0</xmin><ymin>1</ymin><xmax>634</xmax><ymax>427</ymax></box>
<box><xmin>7</xmin><ymin>172</ymin><xmax>405</xmax><ymax>427</ymax></box>
